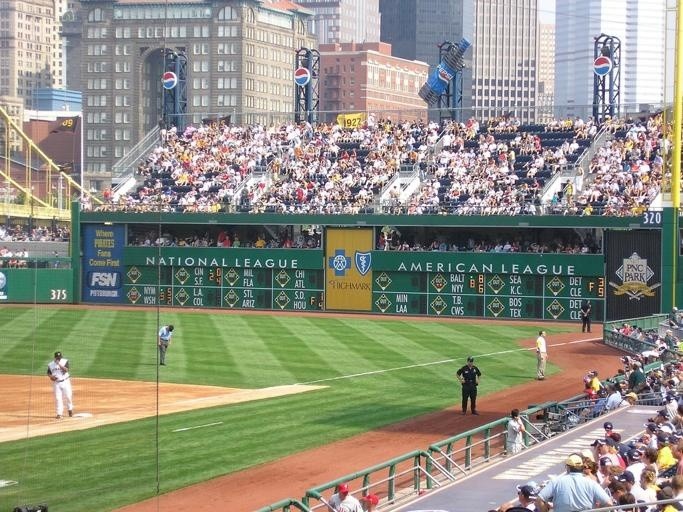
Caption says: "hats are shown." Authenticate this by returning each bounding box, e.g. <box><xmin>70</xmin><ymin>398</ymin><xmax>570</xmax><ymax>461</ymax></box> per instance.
<box><xmin>614</xmin><ymin>470</ymin><xmax>635</xmax><ymax>486</ymax></box>
<box><xmin>600</xmin><ymin>457</ymin><xmax>613</xmax><ymax>466</ymax></box>
<box><xmin>467</xmin><ymin>356</ymin><xmax>473</xmax><ymax>361</ymax></box>
<box><xmin>565</xmin><ymin>454</ymin><xmax>583</xmax><ymax>467</ymax></box>
<box><xmin>590</xmin><ymin>439</ymin><xmax>607</xmax><ymax>446</ymax></box>
<box><xmin>644</xmin><ymin>423</ymin><xmax>656</xmax><ymax>431</ymax></box>
<box><xmin>361</xmin><ymin>494</ymin><xmax>378</xmax><ymax>505</ymax></box>
<box><xmin>603</xmin><ymin>421</ymin><xmax>613</xmax><ymax>431</ymax></box>
<box><xmin>666</xmin><ymin>394</ymin><xmax>676</xmax><ymax>400</ymax></box>
<box><xmin>624</xmin><ymin>449</ymin><xmax>641</xmax><ymax>463</ymax></box>
<box><xmin>657</xmin><ymin>409</ymin><xmax>670</xmax><ymax>421</ymax></box>
<box><xmin>338</xmin><ymin>483</ymin><xmax>349</xmax><ymax>494</ymax></box>
<box><xmin>518</xmin><ymin>485</ymin><xmax>539</xmax><ymax>499</ymax></box>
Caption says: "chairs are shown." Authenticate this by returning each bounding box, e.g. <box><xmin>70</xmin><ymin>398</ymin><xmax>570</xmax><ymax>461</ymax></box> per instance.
<box><xmin>115</xmin><ymin>123</ymin><xmax>671</xmax><ymax>216</ymax></box>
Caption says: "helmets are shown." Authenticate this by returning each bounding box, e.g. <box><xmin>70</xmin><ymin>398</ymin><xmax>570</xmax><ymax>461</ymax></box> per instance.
<box><xmin>55</xmin><ymin>352</ymin><xmax>61</xmax><ymax>358</ymax></box>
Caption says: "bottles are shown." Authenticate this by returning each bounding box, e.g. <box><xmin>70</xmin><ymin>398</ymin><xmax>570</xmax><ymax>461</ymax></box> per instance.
<box><xmin>418</xmin><ymin>37</ymin><xmax>471</xmax><ymax>106</ymax></box>
